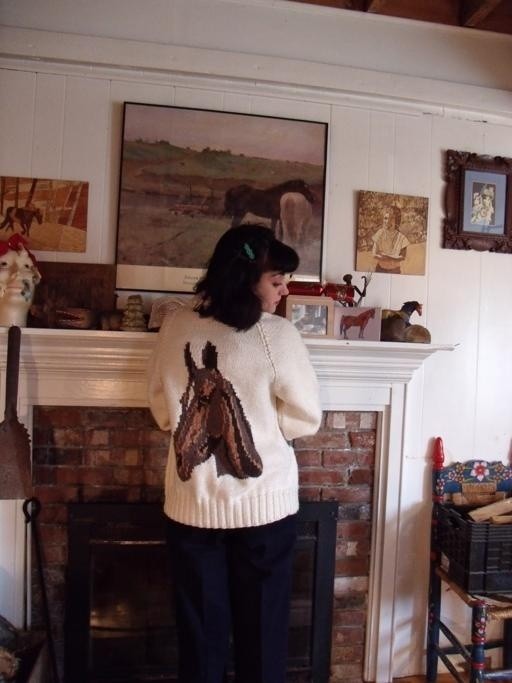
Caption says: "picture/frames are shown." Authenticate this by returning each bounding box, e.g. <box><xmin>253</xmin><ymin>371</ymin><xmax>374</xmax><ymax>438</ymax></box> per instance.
<box><xmin>110</xmin><ymin>98</ymin><xmax>334</xmax><ymax>298</ymax></box>
<box><xmin>441</xmin><ymin>147</ymin><xmax>512</xmax><ymax>255</ymax></box>
<box><xmin>284</xmin><ymin>294</ymin><xmax>336</xmax><ymax>341</ymax></box>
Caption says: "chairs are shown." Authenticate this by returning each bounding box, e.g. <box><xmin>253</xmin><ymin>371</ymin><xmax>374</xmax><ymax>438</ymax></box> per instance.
<box><xmin>421</xmin><ymin>434</ymin><xmax>512</xmax><ymax>681</ymax></box>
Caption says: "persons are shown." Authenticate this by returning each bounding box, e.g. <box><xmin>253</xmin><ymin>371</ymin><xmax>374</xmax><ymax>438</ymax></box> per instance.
<box><xmin>146</xmin><ymin>222</ymin><xmax>326</xmax><ymax>683</ymax></box>
<box><xmin>0</xmin><ymin>231</ymin><xmax>43</xmax><ymax>328</ymax></box>
<box><xmin>370</xmin><ymin>204</ymin><xmax>409</xmax><ymax>273</ymax></box>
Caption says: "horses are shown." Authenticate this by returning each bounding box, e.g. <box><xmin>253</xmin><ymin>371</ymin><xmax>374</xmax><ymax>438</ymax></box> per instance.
<box><xmin>340</xmin><ymin>309</ymin><xmax>375</xmax><ymax>340</ymax></box>
<box><xmin>222</xmin><ymin>179</ymin><xmax>323</xmax><ymax>247</ymax></box>
<box><xmin>381</xmin><ymin>301</ymin><xmax>431</xmax><ymax>343</ymax></box>
<box><xmin>0</xmin><ymin>207</ymin><xmax>43</xmax><ymax>237</ymax></box>
